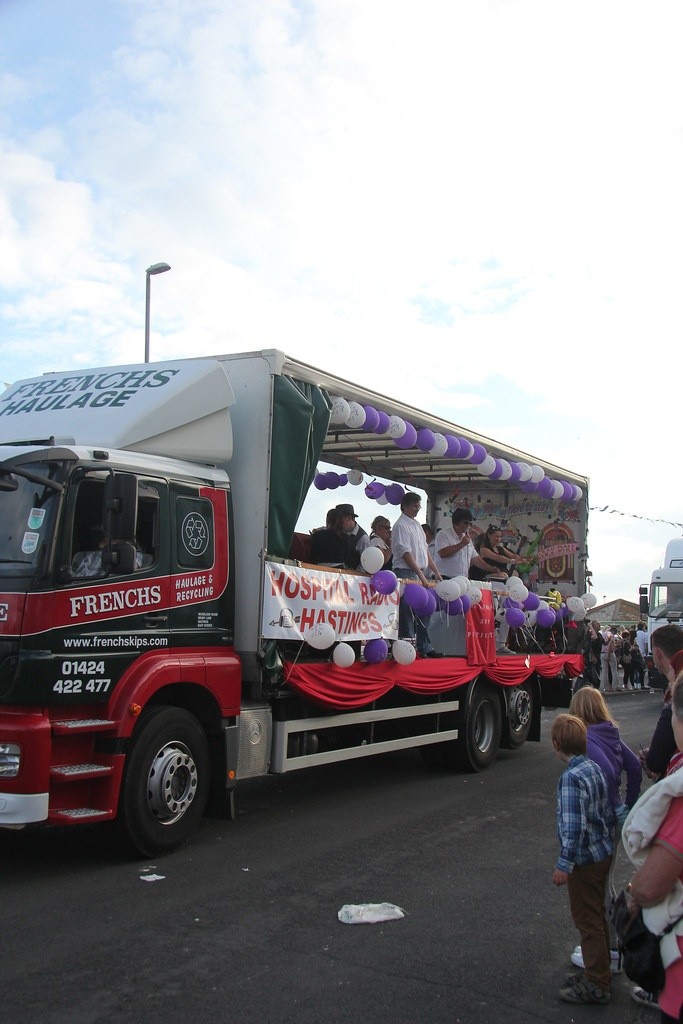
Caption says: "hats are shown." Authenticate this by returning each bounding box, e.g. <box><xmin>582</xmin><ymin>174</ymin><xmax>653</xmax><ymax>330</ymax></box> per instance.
<box><xmin>335</xmin><ymin>504</ymin><xmax>359</xmax><ymax>517</ymax></box>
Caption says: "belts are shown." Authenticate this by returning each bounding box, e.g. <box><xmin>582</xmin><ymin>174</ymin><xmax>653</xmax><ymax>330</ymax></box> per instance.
<box><xmin>601</xmin><ymin>651</ymin><xmax>614</xmax><ymax>653</ymax></box>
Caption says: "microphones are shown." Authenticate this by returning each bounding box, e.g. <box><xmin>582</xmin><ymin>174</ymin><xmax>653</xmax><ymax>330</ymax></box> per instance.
<box><xmin>465</xmin><ymin>528</ymin><xmax>468</xmax><ymax>535</ymax></box>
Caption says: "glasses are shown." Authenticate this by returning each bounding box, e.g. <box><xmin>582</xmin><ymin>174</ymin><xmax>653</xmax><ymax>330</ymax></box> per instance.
<box><xmin>409</xmin><ymin>504</ymin><xmax>422</xmax><ymax>510</ymax></box>
<box><xmin>378</xmin><ymin>526</ymin><xmax>391</xmax><ymax>531</ymax></box>
<box><xmin>492</xmin><ymin>525</ymin><xmax>501</xmax><ymax>529</ymax></box>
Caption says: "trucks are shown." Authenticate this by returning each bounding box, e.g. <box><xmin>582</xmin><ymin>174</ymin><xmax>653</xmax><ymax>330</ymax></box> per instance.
<box><xmin>637</xmin><ymin>537</ymin><xmax>683</xmax><ymax>690</ymax></box>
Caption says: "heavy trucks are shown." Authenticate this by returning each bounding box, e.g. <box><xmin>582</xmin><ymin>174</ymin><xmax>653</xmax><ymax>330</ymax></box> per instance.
<box><xmin>0</xmin><ymin>347</ymin><xmax>593</xmax><ymax>862</ymax></box>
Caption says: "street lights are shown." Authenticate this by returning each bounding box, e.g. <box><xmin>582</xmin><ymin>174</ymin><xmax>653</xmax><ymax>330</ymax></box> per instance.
<box><xmin>145</xmin><ymin>262</ymin><xmax>171</xmax><ymax>364</ymax></box>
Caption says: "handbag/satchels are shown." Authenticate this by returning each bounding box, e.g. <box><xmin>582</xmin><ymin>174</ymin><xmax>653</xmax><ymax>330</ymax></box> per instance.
<box><xmin>621</xmin><ymin>911</ymin><xmax>667</xmax><ymax>993</ymax></box>
<box><xmin>623</xmin><ymin>655</ymin><xmax>632</xmax><ymax>664</ymax></box>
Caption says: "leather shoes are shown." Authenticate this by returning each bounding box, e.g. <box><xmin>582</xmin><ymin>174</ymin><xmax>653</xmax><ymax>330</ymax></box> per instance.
<box><xmin>422</xmin><ymin>649</ymin><xmax>445</xmax><ymax>658</ymax></box>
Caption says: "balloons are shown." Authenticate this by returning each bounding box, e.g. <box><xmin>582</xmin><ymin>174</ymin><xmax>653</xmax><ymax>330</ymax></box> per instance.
<box><xmin>333</xmin><ymin>643</ymin><xmax>355</xmax><ymax>668</ymax></box>
<box><xmin>361</xmin><ymin>547</ymin><xmax>384</xmax><ymax>575</ymax></box>
<box><xmin>305</xmin><ymin>623</ymin><xmax>335</xmax><ymax>650</ymax></box>
<box><xmin>364</xmin><ymin>639</ymin><xmax>388</xmax><ymax>664</ymax></box>
<box><xmin>504</xmin><ymin>576</ymin><xmax>596</xmax><ymax>629</ymax></box>
<box><xmin>435</xmin><ymin>575</ymin><xmax>482</xmax><ymax>616</ymax></box>
<box><xmin>328</xmin><ymin>395</ymin><xmax>582</xmax><ymax>501</ymax></box>
<box><xmin>371</xmin><ymin>570</ymin><xmax>398</xmax><ymax>595</ymax></box>
<box><xmin>403</xmin><ymin>583</ymin><xmax>438</xmax><ymax>618</ymax></box>
<box><xmin>392</xmin><ymin>640</ymin><xmax>416</xmax><ymax>665</ymax></box>
<box><xmin>314</xmin><ymin>467</ymin><xmax>404</xmax><ymax>505</ymax></box>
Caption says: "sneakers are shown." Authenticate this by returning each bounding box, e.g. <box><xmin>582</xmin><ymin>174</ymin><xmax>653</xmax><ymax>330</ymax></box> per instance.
<box><xmin>631</xmin><ymin>987</ymin><xmax>659</xmax><ymax>1007</ymax></box>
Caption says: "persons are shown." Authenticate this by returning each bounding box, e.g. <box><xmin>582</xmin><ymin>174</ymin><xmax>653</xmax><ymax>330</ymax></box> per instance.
<box><xmin>429</xmin><ymin>507</ymin><xmax>501</xmax><ymax>656</ymax></box>
<box><xmin>389</xmin><ymin>492</ymin><xmax>447</xmax><ymax>657</ymax></box>
<box><xmin>368</xmin><ymin>515</ymin><xmax>393</xmax><ymax>571</ymax></box>
<box><xmin>310</xmin><ymin>504</ymin><xmax>370</xmax><ymax>657</ymax></box>
<box><xmin>572</xmin><ymin>618</ymin><xmax>649</xmax><ymax>693</ymax></box>
<box><xmin>478</xmin><ymin>524</ymin><xmax>521</xmax><ymax>654</ymax></box>
<box><xmin>550</xmin><ymin>625</ymin><xmax>682</xmax><ymax>1023</ymax></box>
<box><xmin>76</xmin><ymin>519</ymin><xmax>143</xmax><ymax>576</ymax></box>
<box><xmin>421</xmin><ymin>523</ymin><xmax>436</xmax><ymax>579</ymax></box>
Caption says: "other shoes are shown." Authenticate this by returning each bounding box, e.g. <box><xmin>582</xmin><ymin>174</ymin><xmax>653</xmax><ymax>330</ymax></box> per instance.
<box><xmin>615</xmin><ymin>687</ymin><xmax>622</xmax><ymax>691</ymax></box>
<box><xmin>495</xmin><ymin>646</ymin><xmax>516</xmax><ymax>654</ymax></box>
<box><xmin>571</xmin><ymin>946</ymin><xmax>623</xmax><ymax>973</ymax></box>
<box><xmin>558</xmin><ymin>977</ymin><xmax>611</xmax><ymax>1004</ymax></box>
<box><xmin>632</xmin><ymin>687</ymin><xmax>638</xmax><ymax>690</ymax></box>
<box><xmin>564</xmin><ymin>971</ymin><xmax>585</xmax><ymax>983</ymax></box>
<box><xmin>624</xmin><ymin>687</ymin><xmax>633</xmax><ymax>690</ymax></box>
<box><xmin>641</xmin><ymin>685</ymin><xmax>651</xmax><ymax>689</ymax></box>
